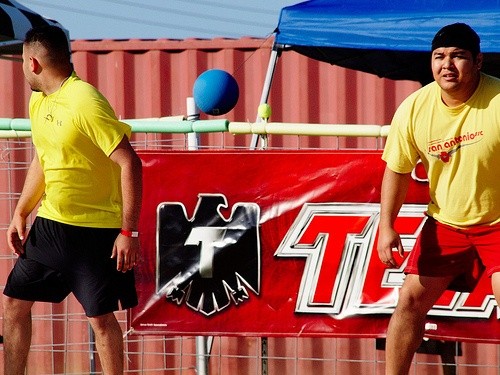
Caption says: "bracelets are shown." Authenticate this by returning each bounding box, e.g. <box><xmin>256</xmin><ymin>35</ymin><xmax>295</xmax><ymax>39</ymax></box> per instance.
<box><xmin>119</xmin><ymin>227</ymin><xmax>140</xmax><ymax>238</ymax></box>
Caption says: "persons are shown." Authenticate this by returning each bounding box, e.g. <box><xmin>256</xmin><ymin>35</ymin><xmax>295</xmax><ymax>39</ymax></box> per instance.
<box><xmin>0</xmin><ymin>25</ymin><xmax>142</xmax><ymax>375</ymax></box>
<box><xmin>377</xmin><ymin>21</ymin><xmax>500</xmax><ymax>374</ymax></box>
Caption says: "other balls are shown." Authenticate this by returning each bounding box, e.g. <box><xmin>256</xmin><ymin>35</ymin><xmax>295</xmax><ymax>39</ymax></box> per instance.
<box><xmin>191</xmin><ymin>69</ymin><xmax>241</xmax><ymax>117</ymax></box>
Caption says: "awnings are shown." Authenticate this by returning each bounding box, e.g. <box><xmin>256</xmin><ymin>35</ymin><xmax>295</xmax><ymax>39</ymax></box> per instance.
<box><xmin>250</xmin><ymin>0</ymin><xmax>500</xmax><ymax>151</ymax></box>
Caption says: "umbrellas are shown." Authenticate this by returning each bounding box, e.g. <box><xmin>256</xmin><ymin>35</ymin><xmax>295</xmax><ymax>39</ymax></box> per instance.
<box><xmin>0</xmin><ymin>0</ymin><xmax>71</xmax><ymax>57</ymax></box>
